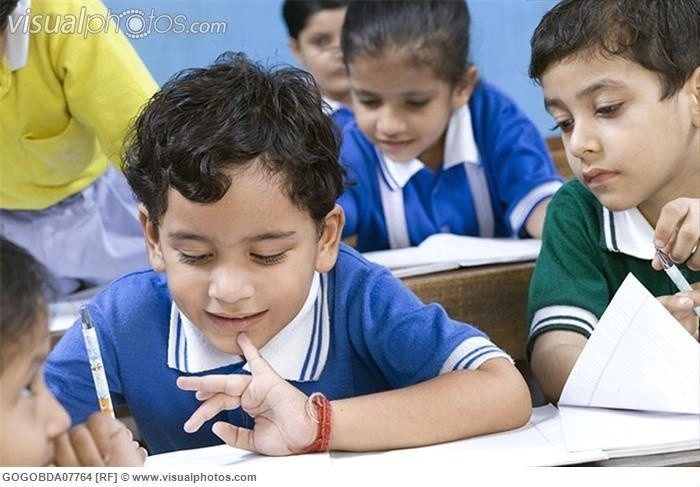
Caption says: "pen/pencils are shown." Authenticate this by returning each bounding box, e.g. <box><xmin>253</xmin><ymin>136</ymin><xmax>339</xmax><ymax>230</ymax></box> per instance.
<box><xmin>80</xmin><ymin>300</ymin><xmax>116</xmax><ymax>420</ymax></box>
<box><xmin>656</xmin><ymin>247</ymin><xmax>700</xmax><ymax>317</ymax></box>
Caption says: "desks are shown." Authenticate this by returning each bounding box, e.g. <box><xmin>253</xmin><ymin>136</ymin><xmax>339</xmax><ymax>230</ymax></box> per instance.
<box><xmin>39</xmin><ymin>257</ymin><xmax>700</xmax><ymax>467</ymax></box>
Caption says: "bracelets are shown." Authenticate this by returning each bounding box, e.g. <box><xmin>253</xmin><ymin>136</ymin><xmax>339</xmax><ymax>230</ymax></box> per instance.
<box><xmin>303</xmin><ymin>390</ymin><xmax>330</xmax><ymax>455</ymax></box>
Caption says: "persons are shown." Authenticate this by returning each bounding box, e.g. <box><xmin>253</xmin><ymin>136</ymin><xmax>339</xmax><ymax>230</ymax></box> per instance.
<box><xmin>519</xmin><ymin>0</ymin><xmax>700</xmax><ymax>407</ymax></box>
<box><xmin>44</xmin><ymin>47</ymin><xmax>533</xmax><ymax>466</ymax></box>
<box><xmin>0</xmin><ymin>0</ymin><xmax>169</xmax><ymax>302</ymax></box>
<box><xmin>0</xmin><ymin>235</ymin><xmax>152</xmax><ymax>466</ymax></box>
<box><xmin>281</xmin><ymin>0</ymin><xmax>356</xmax><ymax>132</ymax></box>
<box><xmin>323</xmin><ymin>1</ymin><xmax>570</xmax><ymax>250</ymax></box>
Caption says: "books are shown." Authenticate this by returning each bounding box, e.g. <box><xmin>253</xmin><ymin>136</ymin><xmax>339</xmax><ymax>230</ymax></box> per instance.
<box><xmin>357</xmin><ymin>232</ymin><xmax>541</xmax><ymax>281</ymax></box>
